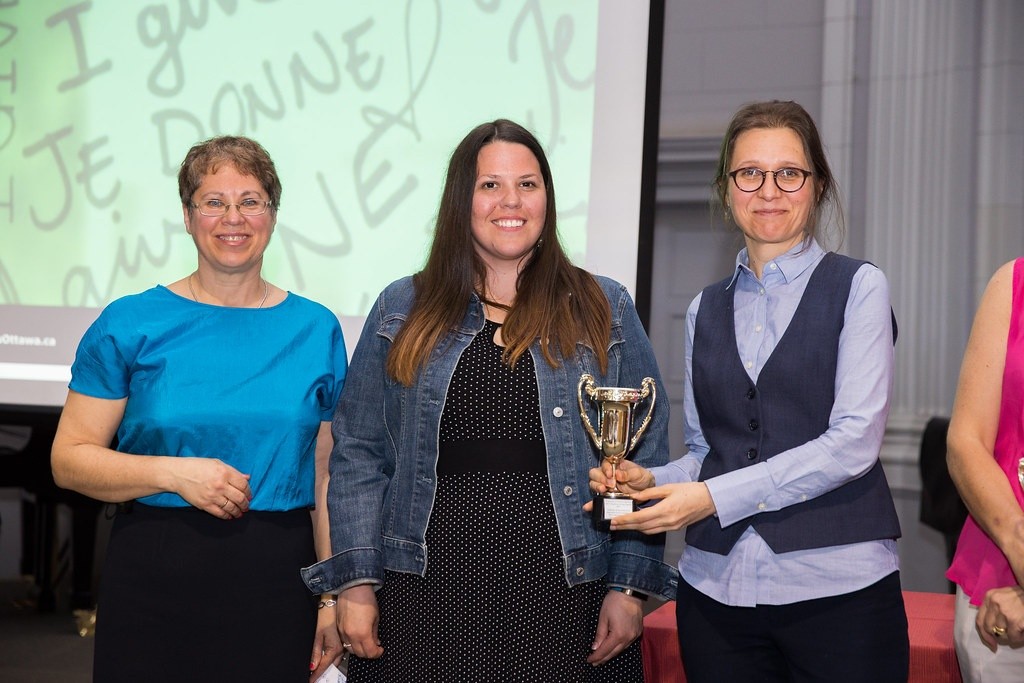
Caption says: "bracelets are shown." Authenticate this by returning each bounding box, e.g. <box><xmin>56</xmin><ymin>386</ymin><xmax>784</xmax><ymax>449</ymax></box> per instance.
<box><xmin>316</xmin><ymin>599</ymin><xmax>337</xmax><ymax>609</ymax></box>
<box><xmin>607</xmin><ymin>585</ymin><xmax>648</xmax><ymax>603</ymax></box>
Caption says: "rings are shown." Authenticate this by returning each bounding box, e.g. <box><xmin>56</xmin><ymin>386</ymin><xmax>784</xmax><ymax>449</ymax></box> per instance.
<box><xmin>991</xmin><ymin>625</ymin><xmax>1005</xmax><ymax>637</ymax></box>
<box><xmin>220</xmin><ymin>498</ymin><xmax>229</xmax><ymax>509</ymax></box>
<box><xmin>342</xmin><ymin>642</ymin><xmax>351</xmax><ymax>649</ymax></box>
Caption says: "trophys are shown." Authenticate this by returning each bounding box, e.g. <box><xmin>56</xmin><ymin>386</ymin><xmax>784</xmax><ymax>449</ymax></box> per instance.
<box><xmin>576</xmin><ymin>374</ymin><xmax>656</xmax><ymax>529</ymax></box>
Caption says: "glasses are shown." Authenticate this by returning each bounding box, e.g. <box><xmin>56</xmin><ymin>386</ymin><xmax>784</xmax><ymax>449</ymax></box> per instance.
<box><xmin>729</xmin><ymin>166</ymin><xmax>814</xmax><ymax>193</ymax></box>
<box><xmin>190</xmin><ymin>199</ymin><xmax>272</xmax><ymax>216</ymax></box>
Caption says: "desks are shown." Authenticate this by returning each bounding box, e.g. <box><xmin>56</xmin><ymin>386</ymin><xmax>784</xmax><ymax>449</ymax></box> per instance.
<box><xmin>640</xmin><ymin>590</ymin><xmax>963</xmax><ymax>683</ymax></box>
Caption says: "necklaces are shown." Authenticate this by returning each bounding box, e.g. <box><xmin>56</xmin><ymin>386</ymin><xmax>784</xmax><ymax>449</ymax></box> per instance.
<box><xmin>188</xmin><ymin>270</ymin><xmax>268</xmax><ymax>309</ymax></box>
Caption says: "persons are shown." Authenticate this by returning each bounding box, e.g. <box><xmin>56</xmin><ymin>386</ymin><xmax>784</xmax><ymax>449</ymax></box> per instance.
<box><xmin>945</xmin><ymin>260</ymin><xmax>1023</xmax><ymax>683</ymax></box>
<box><xmin>301</xmin><ymin>117</ymin><xmax>682</xmax><ymax>683</ymax></box>
<box><xmin>52</xmin><ymin>135</ymin><xmax>344</xmax><ymax>683</ymax></box>
<box><xmin>581</xmin><ymin>98</ymin><xmax>910</xmax><ymax>683</ymax></box>
